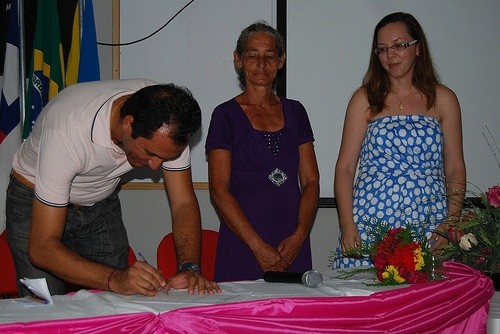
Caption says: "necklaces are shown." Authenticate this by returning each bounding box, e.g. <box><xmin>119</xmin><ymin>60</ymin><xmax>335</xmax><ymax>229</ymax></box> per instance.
<box><xmin>112</xmin><ymin>98</ymin><xmax>127</xmax><ymax>115</ymax></box>
<box><xmin>390</xmin><ymin>88</ymin><xmax>417</xmax><ymax>110</ymax></box>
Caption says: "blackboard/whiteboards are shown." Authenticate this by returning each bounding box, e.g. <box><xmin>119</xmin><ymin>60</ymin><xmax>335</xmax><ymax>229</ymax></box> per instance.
<box><xmin>113</xmin><ymin>0</ymin><xmax>277</xmax><ymax>190</ymax></box>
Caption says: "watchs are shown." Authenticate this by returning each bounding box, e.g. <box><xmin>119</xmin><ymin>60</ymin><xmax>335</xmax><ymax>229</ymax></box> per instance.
<box><xmin>179</xmin><ymin>262</ymin><xmax>201</xmax><ymax>271</ymax></box>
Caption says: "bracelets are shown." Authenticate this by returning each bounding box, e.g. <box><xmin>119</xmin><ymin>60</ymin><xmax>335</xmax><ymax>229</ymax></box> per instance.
<box><xmin>108</xmin><ymin>270</ymin><xmax>116</xmax><ymax>291</ymax></box>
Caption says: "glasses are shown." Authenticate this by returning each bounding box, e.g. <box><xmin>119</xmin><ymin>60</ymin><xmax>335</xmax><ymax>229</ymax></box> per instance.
<box><xmin>375</xmin><ymin>40</ymin><xmax>416</xmax><ymax>55</ymax></box>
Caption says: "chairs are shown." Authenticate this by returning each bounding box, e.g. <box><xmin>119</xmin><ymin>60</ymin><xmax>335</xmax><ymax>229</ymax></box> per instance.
<box><xmin>156</xmin><ymin>230</ymin><xmax>222</xmax><ymax>286</ymax></box>
<box><xmin>0</xmin><ymin>226</ymin><xmax>139</xmax><ymax>301</ymax></box>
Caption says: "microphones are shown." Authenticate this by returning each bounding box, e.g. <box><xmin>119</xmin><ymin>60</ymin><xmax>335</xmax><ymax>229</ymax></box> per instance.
<box><xmin>264</xmin><ymin>270</ymin><xmax>324</xmax><ymax>289</ymax></box>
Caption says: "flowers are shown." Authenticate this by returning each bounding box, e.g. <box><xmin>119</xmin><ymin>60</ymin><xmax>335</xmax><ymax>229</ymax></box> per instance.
<box><xmin>327</xmin><ymin>124</ymin><xmax>500</xmax><ymax>292</ymax></box>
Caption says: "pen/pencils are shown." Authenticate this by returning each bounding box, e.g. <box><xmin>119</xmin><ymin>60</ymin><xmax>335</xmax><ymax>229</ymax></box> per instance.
<box><xmin>138</xmin><ymin>252</ymin><xmax>168</xmax><ymax>295</ymax></box>
<box><xmin>32</xmin><ymin>296</ymin><xmax>47</xmax><ymax>304</ymax></box>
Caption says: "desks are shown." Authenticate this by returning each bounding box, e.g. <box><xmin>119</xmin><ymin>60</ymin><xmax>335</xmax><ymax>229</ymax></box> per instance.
<box><xmin>0</xmin><ymin>275</ymin><xmax>500</xmax><ymax>334</ymax></box>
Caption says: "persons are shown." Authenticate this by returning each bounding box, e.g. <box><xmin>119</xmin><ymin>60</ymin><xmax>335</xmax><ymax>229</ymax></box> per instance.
<box><xmin>334</xmin><ymin>11</ymin><xmax>467</xmax><ymax>268</ymax></box>
<box><xmin>205</xmin><ymin>20</ymin><xmax>320</xmax><ymax>284</ymax></box>
<box><xmin>5</xmin><ymin>79</ymin><xmax>223</xmax><ymax>296</ymax></box>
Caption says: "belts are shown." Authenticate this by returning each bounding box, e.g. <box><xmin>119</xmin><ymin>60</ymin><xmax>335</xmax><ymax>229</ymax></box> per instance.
<box><xmin>13</xmin><ymin>170</ymin><xmax>80</xmax><ymax>210</ymax></box>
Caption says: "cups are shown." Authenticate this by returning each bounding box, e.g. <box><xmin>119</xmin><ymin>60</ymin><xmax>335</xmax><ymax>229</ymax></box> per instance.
<box><xmin>426</xmin><ymin>254</ymin><xmax>443</xmax><ymax>281</ymax></box>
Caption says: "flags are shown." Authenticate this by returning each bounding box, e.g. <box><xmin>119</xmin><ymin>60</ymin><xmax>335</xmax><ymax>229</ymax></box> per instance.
<box><xmin>0</xmin><ymin>0</ymin><xmax>100</xmax><ymax>140</ymax></box>
